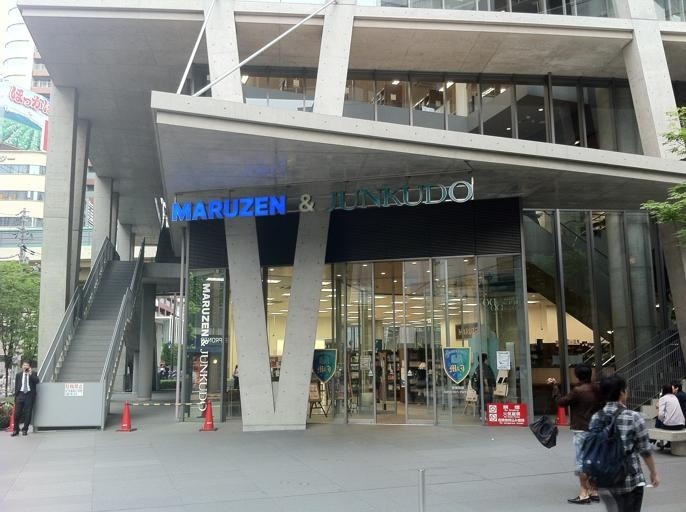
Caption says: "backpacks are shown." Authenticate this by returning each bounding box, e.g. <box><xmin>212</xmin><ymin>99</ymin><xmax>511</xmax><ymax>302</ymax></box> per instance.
<box><xmin>580</xmin><ymin>407</ymin><xmax>636</xmax><ymax>488</ymax></box>
<box><xmin>471</xmin><ymin>364</ymin><xmax>488</xmax><ymax>391</ymax></box>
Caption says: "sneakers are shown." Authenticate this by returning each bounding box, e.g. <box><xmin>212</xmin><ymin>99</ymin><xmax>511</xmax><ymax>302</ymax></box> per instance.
<box><xmin>648</xmin><ymin>438</ymin><xmax>672</xmax><ymax>452</ymax></box>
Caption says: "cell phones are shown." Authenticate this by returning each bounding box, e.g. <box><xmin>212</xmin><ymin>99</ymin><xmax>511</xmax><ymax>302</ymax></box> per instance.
<box><xmin>25</xmin><ymin>368</ymin><xmax>29</xmax><ymax>372</ymax></box>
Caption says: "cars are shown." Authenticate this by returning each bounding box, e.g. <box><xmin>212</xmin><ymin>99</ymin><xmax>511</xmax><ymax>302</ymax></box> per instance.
<box><xmin>156</xmin><ymin>367</ymin><xmax>180</xmax><ymax>378</ymax></box>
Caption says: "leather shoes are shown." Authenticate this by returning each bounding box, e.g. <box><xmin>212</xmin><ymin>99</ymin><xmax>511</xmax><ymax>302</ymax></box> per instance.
<box><xmin>22</xmin><ymin>429</ymin><xmax>26</xmax><ymax>435</ymax></box>
<box><xmin>10</xmin><ymin>427</ymin><xmax>20</xmax><ymax>436</ymax></box>
<box><xmin>567</xmin><ymin>496</ymin><xmax>591</xmax><ymax>505</ymax></box>
<box><xmin>588</xmin><ymin>494</ymin><xmax>600</xmax><ymax>503</ymax></box>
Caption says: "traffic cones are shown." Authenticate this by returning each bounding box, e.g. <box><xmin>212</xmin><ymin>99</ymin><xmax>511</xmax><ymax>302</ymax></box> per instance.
<box><xmin>554</xmin><ymin>405</ymin><xmax>568</xmax><ymax>426</ymax></box>
<box><xmin>197</xmin><ymin>399</ymin><xmax>218</xmax><ymax>433</ymax></box>
<box><xmin>7</xmin><ymin>401</ymin><xmax>15</xmax><ymax>432</ymax></box>
<box><xmin>113</xmin><ymin>399</ymin><xmax>138</xmax><ymax>432</ymax></box>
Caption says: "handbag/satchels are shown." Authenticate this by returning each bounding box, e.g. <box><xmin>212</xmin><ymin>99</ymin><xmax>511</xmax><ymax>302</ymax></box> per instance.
<box><xmin>528</xmin><ymin>414</ymin><xmax>558</xmax><ymax>449</ymax></box>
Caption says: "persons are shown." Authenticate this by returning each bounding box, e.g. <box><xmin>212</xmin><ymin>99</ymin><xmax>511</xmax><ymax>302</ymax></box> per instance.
<box><xmin>10</xmin><ymin>359</ymin><xmax>39</xmax><ymax>436</ymax></box>
<box><xmin>471</xmin><ymin>353</ymin><xmax>496</xmax><ymax>422</ymax></box>
<box><xmin>417</xmin><ymin>359</ymin><xmax>426</xmax><ymax>403</ymax></box>
<box><xmin>546</xmin><ymin>364</ymin><xmax>605</xmax><ymax>504</ymax></box>
<box><xmin>587</xmin><ymin>375</ymin><xmax>660</xmax><ymax>512</ymax></box>
<box><xmin>648</xmin><ymin>384</ymin><xmax>686</xmax><ymax>451</ymax></box>
<box><xmin>375</xmin><ymin>359</ymin><xmax>383</xmax><ymax>403</ymax></box>
<box><xmin>663</xmin><ymin>381</ymin><xmax>686</xmax><ymax>447</ymax></box>
<box><xmin>160</xmin><ymin>363</ymin><xmax>169</xmax><ymax>379</ymax></box>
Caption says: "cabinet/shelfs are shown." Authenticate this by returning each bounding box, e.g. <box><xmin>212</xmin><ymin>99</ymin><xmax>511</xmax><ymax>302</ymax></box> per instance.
<box><xmin>326</xmin><ymin>348</ymin><xmax>470</xmax><ymax>410</ymax></box>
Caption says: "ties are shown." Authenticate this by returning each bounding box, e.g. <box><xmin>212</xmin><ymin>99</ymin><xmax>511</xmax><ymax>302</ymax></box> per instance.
<box><xmin>23</xmin><ymin>374</ymin><xmax>27</xmax><ymax>395</ymax></box>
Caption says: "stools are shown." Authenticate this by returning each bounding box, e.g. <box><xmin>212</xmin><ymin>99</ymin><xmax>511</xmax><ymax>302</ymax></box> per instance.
<box><xmin>648</xmin><ymin>427</ymin><xmax>686</xmax><ymax>456</ymax></box>
